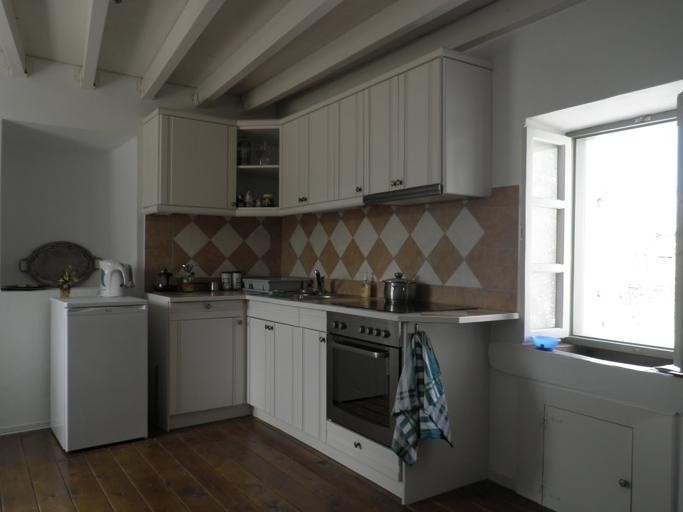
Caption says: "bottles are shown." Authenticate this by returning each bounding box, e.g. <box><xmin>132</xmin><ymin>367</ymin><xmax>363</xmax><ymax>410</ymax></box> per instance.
<box><xmin>238</xmin><ymin>190</ymin><xmax>275</xmax><ymax>207</ymax></box>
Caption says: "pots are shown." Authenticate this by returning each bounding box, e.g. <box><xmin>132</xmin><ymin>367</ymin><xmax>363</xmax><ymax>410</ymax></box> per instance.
<box><xmin>382</xmin><ymin>272</ymin><xmax>417</xmax><ymax>303</ymax></box>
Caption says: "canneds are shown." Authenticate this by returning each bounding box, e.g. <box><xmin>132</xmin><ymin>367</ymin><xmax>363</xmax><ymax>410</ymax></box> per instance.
<box><xmin>261</xmin><ymin>194</ymin><xmax>274</xmax><ymax>207</ymax></box>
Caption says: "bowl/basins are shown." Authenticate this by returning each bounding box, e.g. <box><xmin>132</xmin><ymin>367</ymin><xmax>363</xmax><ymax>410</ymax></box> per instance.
<box><xmin>531</xmin><ymin>336</ymin><xmax>562</xmax><ymax>351</ymax></box>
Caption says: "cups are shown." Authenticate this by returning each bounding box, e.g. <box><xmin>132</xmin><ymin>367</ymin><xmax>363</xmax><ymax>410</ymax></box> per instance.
<box><xmin>221</xmin><ymin>271</ymin><xmax>241</xmax><ymax>290</ymax></box>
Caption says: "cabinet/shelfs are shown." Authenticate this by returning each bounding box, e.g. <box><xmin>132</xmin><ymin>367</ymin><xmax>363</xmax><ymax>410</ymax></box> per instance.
<box><xmin>339</xmin><ymin>90</ymin><xmax>364</xmax><ymax>201</ymax></box>
<box><xmin>1</xmin><ymin>285</ymin><xmax>101</xmax><ymax>436</ymax></box>
<box><xmin>325</xmin><ymin>422</ymin><xmax>403</xmax><ymax>483</ymax></box>
<box><xmin>300</xmin><ymin>327</ymin><xmax>327</xmax><ymax>443</ymax></box>
<box><xmin>169</xmin><ymin>301</ymin><xmax>246</xmax><ymax>418</ymax></box>
<box><xmin>282</xmin><ymin>106</ymin><xmax>329</xmax><ymax>209</ymax></box>
<box><xmin>168</xmin><ymin>115</ymin><xmax>229</xmax><ymax>207</ymax></box>
<box><xmin>48</xmin><ymin>295</ymin><xmax>150</xmax><ymax>450</ymax></box>
<box><xmin>368</xmin><ymin>57</ymin><xmax>442</xmax><ymax>196</ymax></box>
<box><xmin>236</xmin><ymin>127</ymin><xmax>282</xmax><ymax>210</ymax></box>
<box><xmin>246</xmin><ymin>300</ymin><xmax>301</xmax><ymax>429</ymax></box>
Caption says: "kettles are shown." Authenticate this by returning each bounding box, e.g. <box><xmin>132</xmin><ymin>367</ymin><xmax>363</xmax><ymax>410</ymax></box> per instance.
<box><xmin>98</xmin><ymin>260</ymin><xmax>127</xmax><ymax>297</ymax></box>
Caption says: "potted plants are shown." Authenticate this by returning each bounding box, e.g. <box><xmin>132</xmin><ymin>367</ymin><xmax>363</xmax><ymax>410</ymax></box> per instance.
<box><xmin>57</xmin><ymin>265</ymin><xmax>77</xmax><ymax>299</ymax></box>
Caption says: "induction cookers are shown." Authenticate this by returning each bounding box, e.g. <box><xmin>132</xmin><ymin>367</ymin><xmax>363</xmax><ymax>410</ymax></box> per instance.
<box><xmin>331</xmin><ymin>297</ymin><xmax>479</xmax><ymax>314</ymax></box>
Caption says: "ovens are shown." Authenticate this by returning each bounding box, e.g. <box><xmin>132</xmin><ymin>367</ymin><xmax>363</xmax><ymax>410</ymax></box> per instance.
<box><xmin>325</xmin><ymin>311</ymin><xmax>404</xmax><ymax>450</ymax></box>
